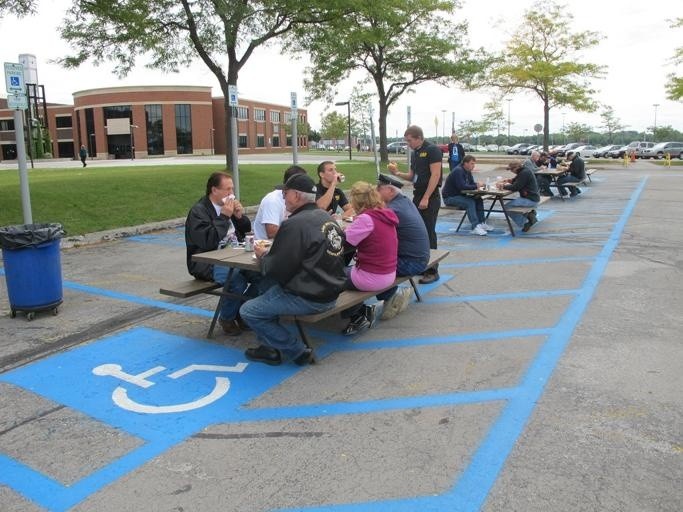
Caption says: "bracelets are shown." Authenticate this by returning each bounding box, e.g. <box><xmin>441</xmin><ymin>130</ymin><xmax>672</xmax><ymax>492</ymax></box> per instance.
<box><xmin>394</xmin><ymin>170</ymin><xmax>400</xmax><ymax>175</ymax></box>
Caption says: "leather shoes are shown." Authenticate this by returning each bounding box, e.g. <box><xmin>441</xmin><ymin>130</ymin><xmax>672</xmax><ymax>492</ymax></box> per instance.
<box><xmin>244</xmin><ymin>343</ymin><xmax>282</xmax><ymax>367</ymax></box>
<box><xmin>418</xmin><ymin>271</ymin><xmax>440</xmax><ymax>285</ymax></box>
<box><xmin>292</xmin><ymin>351</ymin><xmax>314</xmax><ymax>368</ymax></box>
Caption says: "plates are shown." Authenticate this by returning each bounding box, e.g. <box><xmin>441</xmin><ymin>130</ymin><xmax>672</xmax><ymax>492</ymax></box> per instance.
<box><xmin>241</xmin><ymin>239</ymin><xmax>272</xmax><ymax>246</ymax></box>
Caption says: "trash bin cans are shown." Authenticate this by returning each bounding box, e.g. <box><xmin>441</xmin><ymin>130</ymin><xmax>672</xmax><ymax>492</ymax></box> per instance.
<box><xmin>0</xmin><ymin>222</ymin><xmax>67</xmax><ymax>322</ymax></box>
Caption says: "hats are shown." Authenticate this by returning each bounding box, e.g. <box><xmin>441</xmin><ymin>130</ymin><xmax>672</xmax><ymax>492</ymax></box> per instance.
<box><xmin>377</xmin><ymin>172</ymin><xmax>405</xmax><ymax>189</ymax></box>
<box><xmin>540</xmin><ymin>152</ymin><xmax>551</xmax><ymax>158</ymax></box>
<box><xmin>505</xmin><ymin>159</ymin><xmax>523</xmax><ymax>171</ymax></box>
<box><xmin>274</xmin><ymin>172</ymin><xmax>320</xmax><ymax>195</ymax></box>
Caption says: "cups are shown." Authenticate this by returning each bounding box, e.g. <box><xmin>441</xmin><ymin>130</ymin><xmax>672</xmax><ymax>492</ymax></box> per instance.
<box><xmin>499</xmin><ymin>183</ymin><xmax>503</xmax><ymax>191</ymax></box>
<box><xmin>483</xmin><ymin>184</ymin><xmax>489</xmax><ymax>190</ymax></box>
<box><xmin>337</xmin><ymin>175</ymin><xmax>344</xmax><ymax>183</ymax></box>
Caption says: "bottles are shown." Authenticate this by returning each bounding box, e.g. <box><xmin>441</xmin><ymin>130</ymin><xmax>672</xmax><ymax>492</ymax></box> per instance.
<box><xmin>334</xmin><ymin>210</ymin><xmax>342</xmax><ymax>227</ymax></box>
<box><xmin>548</xmin><ymin>163</ymin><xmax>550</xmax><ymax>170</ymax></box>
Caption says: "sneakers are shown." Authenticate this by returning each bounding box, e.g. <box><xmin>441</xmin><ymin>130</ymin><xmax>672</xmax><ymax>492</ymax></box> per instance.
<box><xmin>342</xmin><ymin>304</ymin><xmax>377</xmax><ymax>337</ymax></box>
<box><xmin>379</xmin><ymin>286</ymin><xmax>415</xmax><ymax>321</ymax></box>
<box><xmin>471</xmin><ymin>221</ymin><xmax>495</xmax><ymax>236</ymax></box>
<box><xmin>521</xmin><ymin>209</ymin><xmax>539</xmax><ymax>233</ymax></box>
<box><xmin>218</xmin><ymin>310</ymin><xmax>254</xmax><ymax>337</ymax></box>
<box><xmin>569</xmin><ymin>188</ymin><xmax>581</xmax><ymax>197</ymax></box>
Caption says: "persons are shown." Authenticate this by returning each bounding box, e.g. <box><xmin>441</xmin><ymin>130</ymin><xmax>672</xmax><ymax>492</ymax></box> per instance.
<box><xmin>522</xmin><ymin>148</ymin><xmax>586</xmax><ymax>200</ymax></box>
<box><xmin>330</xmin><ymin>180</ymin><xmax>400</xmax><ymax>337</ymax></box>
<box><xmin>252</xmin><ymin>166</ymin><xmax>307</xmax><ymax>243</ymax></box>
<box><xmin>446</xmin><ymin>134</ymin><xmax>464</xmax><ymax>171</ymax></box>
<box><xmin>184</xmin><ymin>170</ymin><xmax>265</xmax><ymax>338</ymax></box>
<box><xmin>386</xmin><ymin>125</ymin><xmax>442</xmax><ymax>285</ymax></box>
<box><xmin>314</xmin><ymin>160</ymin><xmax>352</xmax><ymax>221</ymax></box>
<box><xmin>372</xmin><ymin>173</ymin><xmax>432</xmax><ymax>322</ymax></box>
<box><xmin>495</xmin><ymin>158</ymin><xmax>540</xmax><ymax>232</ymax></box>
<box><xmin>237</xmin><ymin>172</ymin><xmax>346</xmax><ymax>367</ymax></box>
<box><xmin>440</xmin><ymin>154</ymin><xmax>495</xmax><ymax>236</ymax></box>
<box><xmin>78</xmin><ymin>145</ymin><xmax>87</xmax><ymax>169</ymax></box>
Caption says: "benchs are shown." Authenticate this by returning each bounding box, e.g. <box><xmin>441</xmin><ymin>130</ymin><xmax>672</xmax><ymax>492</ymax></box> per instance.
<box><xmin>276</xmin><ymin>247</ymin><xmax>450</xmax><ymax>362</ymax></box>
<box><xmin>157</xmin><ymin>276</ymin><xmax>227</xmax><ymax>337</ymax></box>
<box><xmin>439</xmin><ymin>150</ymin><xmax>596</xmax><ymax>237</ymax></box>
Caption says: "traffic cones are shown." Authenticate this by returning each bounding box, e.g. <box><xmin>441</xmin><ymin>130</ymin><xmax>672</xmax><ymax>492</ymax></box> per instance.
<box><xmin>629</xmin><ymin>151</ymin><xmax>637</xmax><ymax>162</ymax></box>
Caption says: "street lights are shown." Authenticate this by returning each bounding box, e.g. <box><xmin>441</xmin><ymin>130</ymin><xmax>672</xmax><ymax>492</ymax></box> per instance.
<box><xmin>441</xmin><ymin>110</ymin><xmax>447</xmax><ymax>144</ymax></box>
<box><xmin>652</xmin><ymin>104</ymin><xmax>660</xmax><ymax>135</ymax></box>
<box><xmin>27</xmin><ymin>117</ymin><xmax>38</xmax><ymax>168</ymax></box>
<box><xmin>505</xmin><ymin>98</ymin><xmax>514</xmax><ymax>146</ymax></box>
<box><xmin>209</xmin><ymin>127</ymin><xmax>215</xmax><ymax>156</ymax></box>
<box><xmin>89</xmin><ymin>134</ymin><xmax>96</xmax><ymax>160</ymax></box>
<box><xmin>560</xmin><ymin>113</ymin><xmax>566</xmax><ymax>145</ymax></box>
<box><xmin>129</xmin><ymin>125</ymin><xmax>139</xmax><ymax>160</ymax></box>
<box><xmin>334</xmin><ymin>100</ymin><xmax>352</xmax><ymax>161</ymax></box>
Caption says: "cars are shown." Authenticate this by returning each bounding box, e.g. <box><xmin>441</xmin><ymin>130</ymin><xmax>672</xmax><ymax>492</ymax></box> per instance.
<box><xmin>437</xmin><ymin>140</ymin><xmax>683</xmax><ymax>159</ymax></box>
<box><xmin>315</xmin><ymin>142</ymin><xmax>419</xmax><ymax>155</ymax></box>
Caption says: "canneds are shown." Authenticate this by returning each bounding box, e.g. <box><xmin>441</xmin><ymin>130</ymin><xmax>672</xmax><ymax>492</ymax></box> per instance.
<box><xmin>245</xmin><ymin>232</ymin><xmax>254</xmax><ymax>252</ymax></box>
<box><xmin>337</xmin><ymin>175</ymin><xmax>345</xmax><ymax>182</ymax></box>
